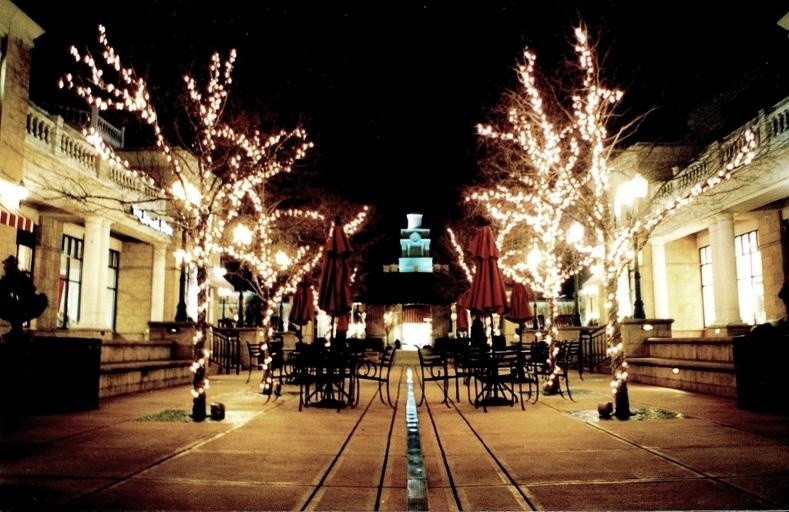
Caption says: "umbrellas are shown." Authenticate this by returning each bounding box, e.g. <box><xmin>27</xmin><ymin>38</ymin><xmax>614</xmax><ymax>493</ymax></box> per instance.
<box><xmin>455</xmin><ymin>294</ymin><xmax>469</xmax><ymax>338</ymax></box>
<box><xmin>504</xmin><ymin>281</ymin><xmax>534</xmax><ymax>345</ymax></box>
<box><xmin>288</xmin><ymin>273</ymin><xmax>315</xmax><ymax>343</ymax></box>
<box><xmin>337</xmin><ymin>314</ymin><xmax>348</xmax><ymax>332</ymax></box>
<box><xmin>468</xmin><ymin>227</ymin><xmax>511</xmax><ymax>354</ymax></box>
<box><xmin>317</xmin><ymin>214</ymin><xmax>354</xmax><ymax>342</ymax></box>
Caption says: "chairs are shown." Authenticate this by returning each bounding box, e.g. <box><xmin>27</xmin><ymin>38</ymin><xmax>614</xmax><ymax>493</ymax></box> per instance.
<box><xmin>243</xmin><ymin>332</ymin><xmax>399</xmax><ymax>412</ymax></box>
<box><xmin>412</xmin><ymin>333</ymin><xmax>584</xmax><ymax>411</ymax></box>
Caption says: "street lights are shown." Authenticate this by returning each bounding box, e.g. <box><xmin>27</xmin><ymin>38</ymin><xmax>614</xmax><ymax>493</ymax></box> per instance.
<box><xmin>566</xmin><ymin>219</ymin><xmax>586</xmax><ymax>326</ymax></box>
<box><xmin>277</xmin><ymin>252</ymin><xmax>291</xmax><ymax>330</ymax></box>
<box><xmin>616</xmin><ymin>173</ymin><xmax>649</xmax><ymax>319</ymax></box>
<box><xmin>231</xmin><ymin>223</ymin><xmax>253</xmax><ymax>327</ymax></box>
<box><xmin>528</xmin><ymin>247</ymin><xmax>540</xmax><ymax>327</ymax></box>
<box><xmin>170</xmin><ymin>181</ymin><xmax>202</xmax><ymax>323</ymax></box>
